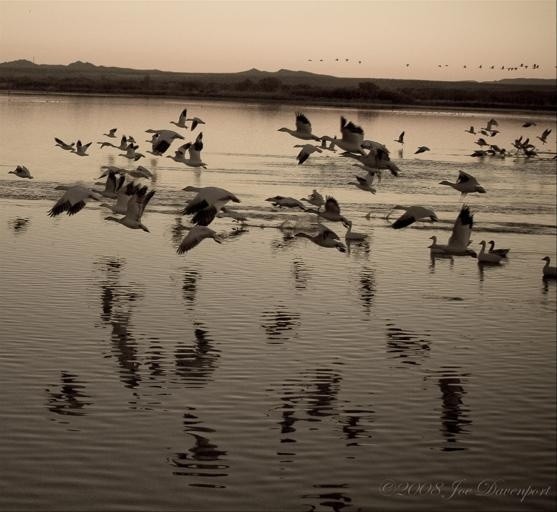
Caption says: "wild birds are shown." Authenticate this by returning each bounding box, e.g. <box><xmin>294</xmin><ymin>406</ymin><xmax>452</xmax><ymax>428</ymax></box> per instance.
<box><xmin>541</xmin><ymin>255</ymin><xmax>556</xmax><ymax>277</ymax></box>
<box><xmin>264</xmin><ymin>111</ymin><xmax>511</xmax><ymax>263</ymax></box>
<box><xmin>188</xmin><ymin>116</ymin><xmax>206</xmax><ymax>131</ymax></box>
<box><xmin>170</xmin><ymin>109</ymin><xmax>188</xmax><ymax>129</ymax></box>
<box><xmin>45</xmin><ymin>127</ymin><xmax>248</xmax><ymax>255</ymax></box>
<box><xmin>7</xmin><ymin>164</ymin><xmax>34</xmax><ymax>180</ymax></box>
<box><xmin>464</xmin><ymin>117</ymin><xmax>552</xmax><ymax>164</ymax></box>
